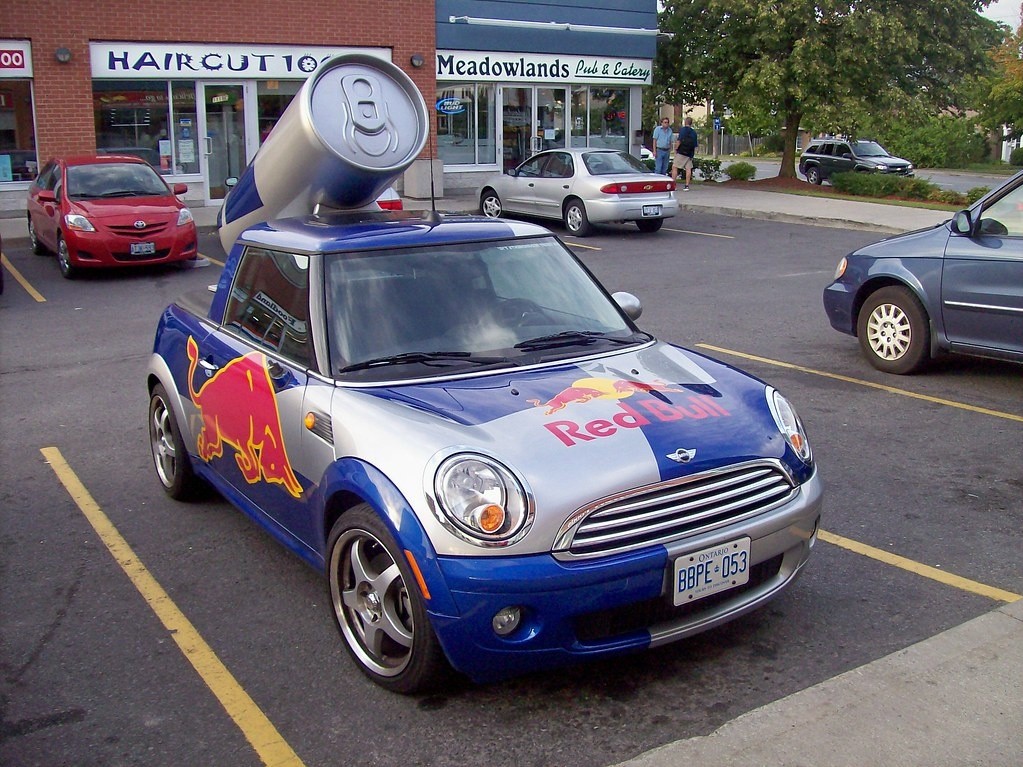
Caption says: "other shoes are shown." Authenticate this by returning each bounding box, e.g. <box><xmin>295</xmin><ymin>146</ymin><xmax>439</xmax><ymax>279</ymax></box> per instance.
<box><xmin>683</xmin><ymin>185</ymin><xmax>689</xmax><ymax>191</ymax></box>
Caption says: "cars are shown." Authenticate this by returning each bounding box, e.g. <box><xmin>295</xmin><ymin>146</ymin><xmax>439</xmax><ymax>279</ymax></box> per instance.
<box><xmin>377</xmin><ymin>187</ymin><xmax>403</xmax><ymax>210</ymax></box>
<box><xmin>821</xmin><ymin>171</ymin><xmax>1023</xmax><ymax>375</ymax></box>
<box><xmin>145</xmin><ymin>52</ymin><xmax>824</xmax><ymax>696</ymax></box>
<box><xmin>97</xmin><ymin>148</ymin><xmax>187</xmax><ymax>175</ymax></box>
<box><xmin>640</xmin><ymin>145</ymin><xmax>655</xmax><ymax>161</ymax></box>
<box><xmin>0</xmin><ymin>150</ymin><xmax>37</xmax><ymax>180</ymax></box>
<box><xmin>25</xmin><ymin>154</ymin><xmax>198</xmax><ymax>279</ymax></box>
<box><xmin>475</xmin><ymin>147</ymin><xmax>679</xmax><ymax>238</ymax></box>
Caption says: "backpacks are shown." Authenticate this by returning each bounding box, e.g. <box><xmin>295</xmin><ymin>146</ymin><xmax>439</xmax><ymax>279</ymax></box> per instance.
<box><xmin>680</xmin><ymin>127</ymin><xmax>694</xmax><ymax>153</ymax></box>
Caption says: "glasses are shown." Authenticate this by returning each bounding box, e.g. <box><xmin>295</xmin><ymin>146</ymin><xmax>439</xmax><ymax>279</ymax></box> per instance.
<box><xmin>662</xmin><ymin>121</ymin><xmax>669</xmax><ymax>123</ymax></box>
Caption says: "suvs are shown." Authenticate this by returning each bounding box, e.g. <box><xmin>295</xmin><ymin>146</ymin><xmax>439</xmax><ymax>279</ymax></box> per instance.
<box><xmin>799</xmin><ymin>138</ymin><xmax>914</xmax><ymax>185</ymax></box>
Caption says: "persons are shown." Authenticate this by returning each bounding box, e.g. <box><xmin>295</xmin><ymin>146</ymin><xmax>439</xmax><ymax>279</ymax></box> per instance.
<box><xmin>672</xmin><ymin>117</ymin><xmax>698</xmax><ymax>191</ymax></box>
<box><xmin>653</xmin><ymin>118</ymin><xmax>673</xmax><ymax>175</ymax></box>
<box><xmin>261</xmin><ymin>122</ymin><xmax>273</xmax><ymax>142</ymax></box>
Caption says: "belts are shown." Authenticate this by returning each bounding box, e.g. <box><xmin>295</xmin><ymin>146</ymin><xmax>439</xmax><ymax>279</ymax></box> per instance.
<box><xmin>657</xmin><ymin>147</ymin><xmax>670</xmax><ymax>151</ymax></box>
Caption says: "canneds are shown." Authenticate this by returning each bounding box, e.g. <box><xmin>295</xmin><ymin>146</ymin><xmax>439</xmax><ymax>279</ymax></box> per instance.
<box><xmin>217</xmin><ymin>51</ymin><xmax>429</xmax><ymax>256</ymax></box>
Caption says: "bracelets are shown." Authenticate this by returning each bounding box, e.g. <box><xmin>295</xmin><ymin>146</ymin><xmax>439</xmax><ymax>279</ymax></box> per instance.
<box><xmin>675</xmin><ymin>149</ymin><xmax>677</xmax><ymax>151</ymax></box>
